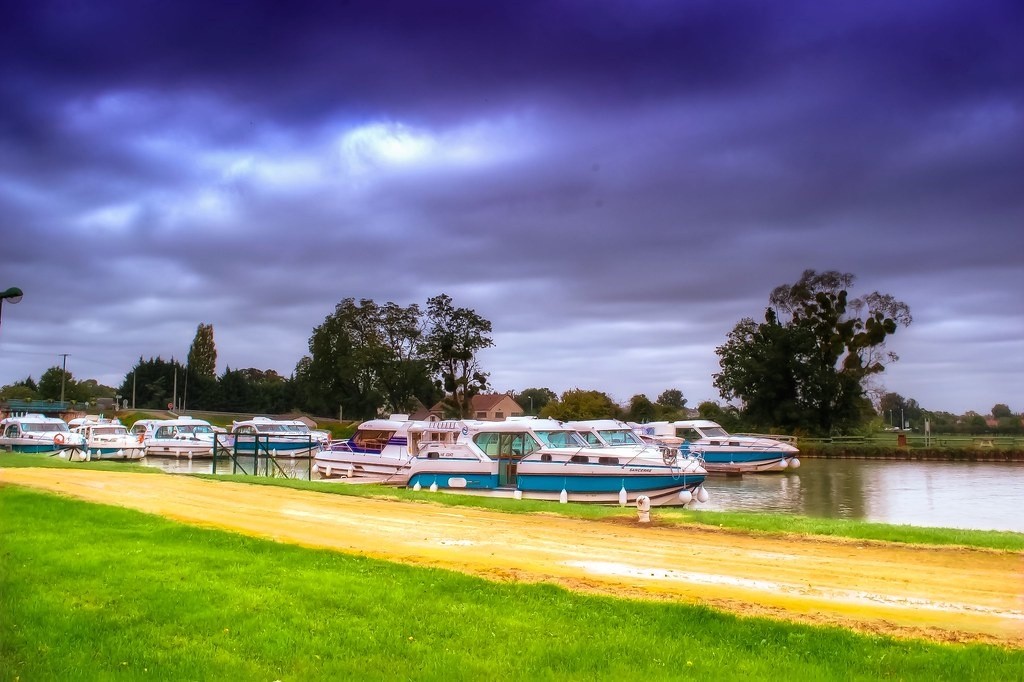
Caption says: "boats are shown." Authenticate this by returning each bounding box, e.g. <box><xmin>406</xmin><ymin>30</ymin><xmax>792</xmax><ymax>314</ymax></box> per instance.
<box><xmin>0</xmin><ymin>414</ymin><xmax>90</xmax><ymax>462</ymax></box>
<box><xmin>130</xmin><ymin>416</ymin><xmax>333</xmax><ymax>460</ymax></box>
<box><xmin>68</xmin><ymin>414</ymin><xmax>146</xmax><ymax>462</ymax></box>
<box><xmin>314</xmin><ymin>414</ymin><xmax>800</xmax><ymax>508</ymax></box>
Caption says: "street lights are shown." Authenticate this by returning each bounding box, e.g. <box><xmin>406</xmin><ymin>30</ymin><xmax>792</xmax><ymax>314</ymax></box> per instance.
<box><xmin>890</xmin><ymin>410</ymin><xmax>892</xmax><ymax>426</ymax></box>
<box><xmin>528</xmin><ymin>396</ymin><xmax>532</xmax><ymax>410</ymax></box>
<box><xmin>0</xmin><ymin>287</ymin><xmax>23</xmax><ymax>325</ymax></box>
<box><xmin>901</xmin><ymin>409</ymin><xmax>903</xmax><ymax>429</ymax></box>
